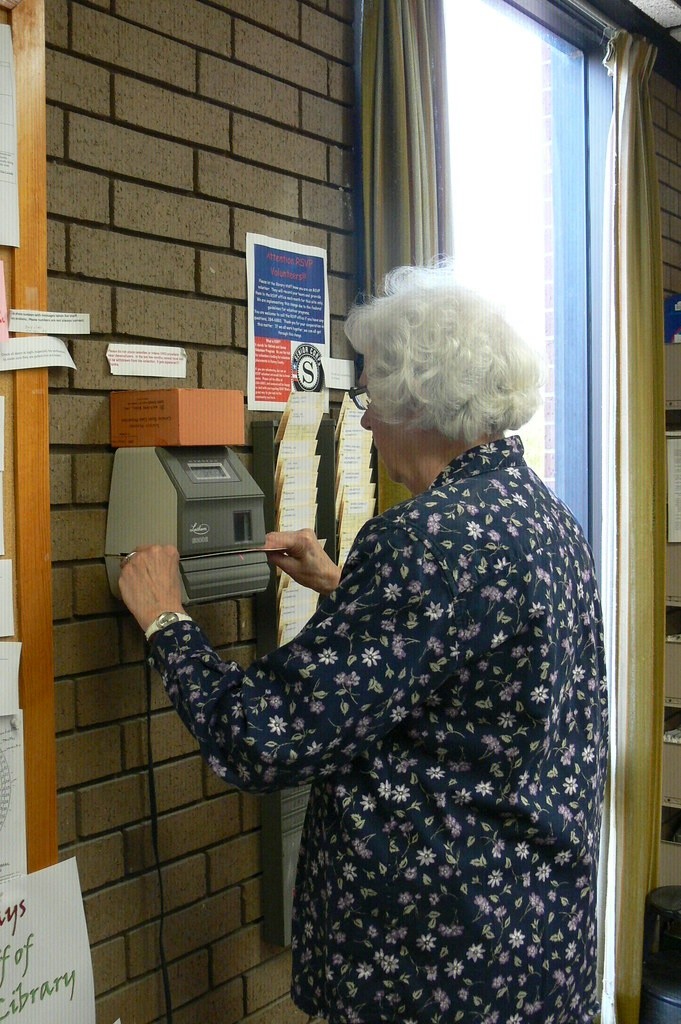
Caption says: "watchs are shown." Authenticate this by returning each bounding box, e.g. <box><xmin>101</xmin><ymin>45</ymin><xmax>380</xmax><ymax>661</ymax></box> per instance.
<box><xmin>145</xmin><ymin>612</ymin><xmax>195</xmax><ymax>641</ymax></box>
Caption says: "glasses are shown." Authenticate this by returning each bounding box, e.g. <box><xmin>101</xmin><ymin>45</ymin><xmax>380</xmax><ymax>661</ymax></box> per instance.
<box><xmin>347</xmin><ymin>384</ymin><xmax>369</xmax><ymax>409</ymax></box>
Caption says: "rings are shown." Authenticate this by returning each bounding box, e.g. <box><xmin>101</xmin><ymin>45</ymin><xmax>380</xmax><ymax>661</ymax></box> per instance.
<box><xmin>120</xmin><ymin>551</ymin><xmax>136</xmax><ymax>567</ymax></box>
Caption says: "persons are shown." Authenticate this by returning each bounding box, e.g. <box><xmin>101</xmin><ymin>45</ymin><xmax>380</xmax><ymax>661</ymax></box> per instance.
<box><xmin>118</xmin><ymin>264</ymin><xmax>609</xmax><ymax>1024</ymax></box>
